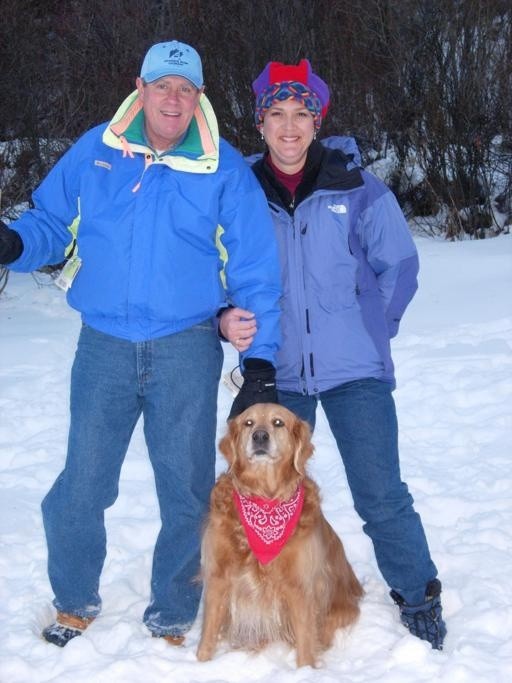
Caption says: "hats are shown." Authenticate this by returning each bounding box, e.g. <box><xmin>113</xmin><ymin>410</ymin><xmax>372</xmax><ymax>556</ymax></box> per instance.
<box><xmin>140</xmin><ymin>39</ymin><xmax>206</xmax><ymax>89</ymax></box>
<box><xmin>251</xmin><ymin>58</ymin><xmax>331</xmax><ymax>133</ymax></box>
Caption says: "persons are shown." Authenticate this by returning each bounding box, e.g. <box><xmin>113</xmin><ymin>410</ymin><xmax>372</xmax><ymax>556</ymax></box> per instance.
<box><xmin>208</xmin><ymin>56</ymin><xmax>448</xmax><ymax>650</ymax></box>
<box><xmin>0</xmin><ymin>39</ymin><xmax>286</xmax><ymax>648</ymax></box>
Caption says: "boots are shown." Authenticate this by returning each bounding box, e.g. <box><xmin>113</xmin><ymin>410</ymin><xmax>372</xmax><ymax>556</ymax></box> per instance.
<box><xmin>390</xmin><ymin>577</ymin><xmax>447</xmax><ymax>651</ymax></box>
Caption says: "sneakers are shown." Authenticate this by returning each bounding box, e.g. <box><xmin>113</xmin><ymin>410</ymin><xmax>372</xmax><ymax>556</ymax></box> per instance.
<box><xmin>150</xmin><ymin>628</ymin><xmax>187</xmax><ymax>647</ymax></box>
<box><xmin>42</xmin><ymin>608</ymin><xmax>96</xmax><ymax>647</ymax></box>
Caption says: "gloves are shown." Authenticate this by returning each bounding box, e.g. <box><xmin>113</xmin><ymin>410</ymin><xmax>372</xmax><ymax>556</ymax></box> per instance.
<box><xmin>227</xmin><ymin>357</ymin><xmax>279</xmax><ymax>419</ymax></box>
<box><xmin>0</xmin><ymin>220</ymin><xmax>24</xmax><ymax>266</ymax></box>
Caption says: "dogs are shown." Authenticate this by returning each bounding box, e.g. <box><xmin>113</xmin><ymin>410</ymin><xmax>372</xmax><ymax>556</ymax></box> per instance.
<box><xmin>192</xmin><ymin>402</ymin><xmax>367</xmax><ymax>670</ymax></box>
